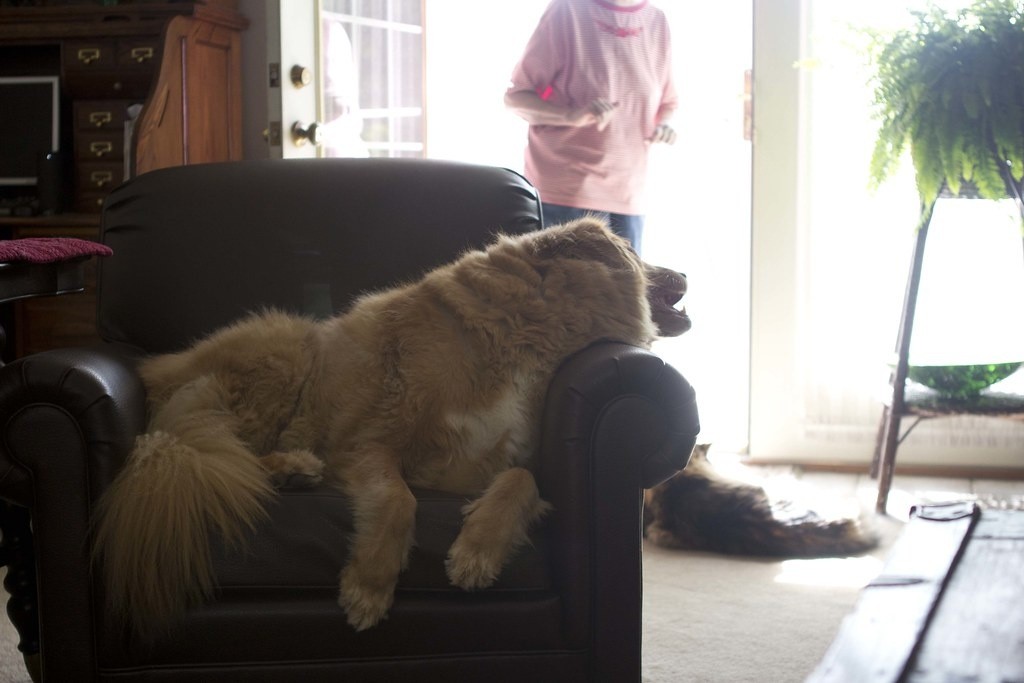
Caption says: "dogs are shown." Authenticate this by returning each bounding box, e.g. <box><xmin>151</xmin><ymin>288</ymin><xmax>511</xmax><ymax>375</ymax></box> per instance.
<box><xmin>94</xmin><ymin>208</ymin><xmax>692</xmax><ymax>634</ymax></box>
<box><xmin>643</xmin><ymin>442</ymin><xmax>886</xmax><ymax>559</ymax></box>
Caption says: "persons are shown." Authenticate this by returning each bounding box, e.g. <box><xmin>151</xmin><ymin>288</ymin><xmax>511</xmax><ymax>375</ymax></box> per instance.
<box><xmin>504</xmin><ymin>1</ymin><xmax>684</xmax><ymax>272</ymax></box>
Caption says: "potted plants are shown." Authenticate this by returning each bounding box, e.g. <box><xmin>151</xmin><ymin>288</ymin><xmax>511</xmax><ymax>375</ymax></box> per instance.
<box><xmin>862</xmin><ymin>0</ymin><xmax>1024</xmax><ymax>212</ymax></box>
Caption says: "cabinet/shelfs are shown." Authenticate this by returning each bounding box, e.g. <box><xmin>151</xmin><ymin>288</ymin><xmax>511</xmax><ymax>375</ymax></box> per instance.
<box><xmin>0</xmin><ymin>0</ymin><xmax>252</xmax><ymax>358</ymax></box>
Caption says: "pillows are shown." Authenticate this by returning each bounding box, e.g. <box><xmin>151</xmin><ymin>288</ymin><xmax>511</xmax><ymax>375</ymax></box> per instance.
<box><xmin>0</xmin><ymin>236</ymin><xmax>113</xmax><ymax>263</ymax></box>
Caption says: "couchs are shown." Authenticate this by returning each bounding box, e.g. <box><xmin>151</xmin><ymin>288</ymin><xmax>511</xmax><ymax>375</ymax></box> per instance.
<box><xmin>0</xmin><ymin>155</ymin><xmax>701</xmax><ymax>683</ymax></box>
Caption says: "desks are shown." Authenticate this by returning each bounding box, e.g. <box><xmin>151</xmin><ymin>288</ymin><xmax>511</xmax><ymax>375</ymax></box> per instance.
<box><xmin>870</xmin><ymin>167</ymin><xmax>1024</xmax><ymax>516</ymax></box>
